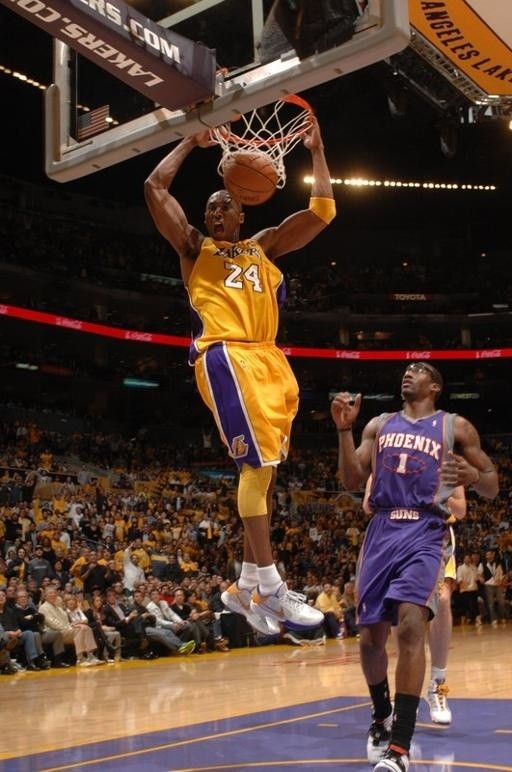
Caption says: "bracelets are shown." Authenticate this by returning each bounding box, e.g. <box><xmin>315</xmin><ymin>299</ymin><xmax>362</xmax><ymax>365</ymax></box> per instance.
<box><xmin>337</xmin><ymin>428</ymin><xmax>352</xmax><ymax>431</ymax></box>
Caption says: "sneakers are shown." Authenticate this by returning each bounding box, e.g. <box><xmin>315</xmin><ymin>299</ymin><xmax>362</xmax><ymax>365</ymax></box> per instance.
<box><xmin>367</xmin><ymin>702</ymin><xmax>397</xmax><ymax>765</ymax></box>
<box><xmin>219</xmin><ymin>580</ymin><xmax>283</xmax><ymax>638</ymax></box>
<box><xmin>250</xmin><ymin>583</ymin><xmax>325</xmax><ymax>632</ymax></box>
<box><xmin>0</xmin><ymin>638</ymin><xmax>231</xmax><ymax>675</ymax></box>
<box><xmin>373</xmin><ymin>739</ymin><xmax>416</xmax><ymax>772</ymax></box>
<box><xmin>423</xmin><ymin>679</ymin><xmax>452</xmax><ymax>725</ymax></box>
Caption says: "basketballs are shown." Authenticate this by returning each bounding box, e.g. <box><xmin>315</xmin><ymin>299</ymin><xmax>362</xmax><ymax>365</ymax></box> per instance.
<box><xmin>223</xmin><ymin>150</ymin><xmax>276</xmax><ymax>205</ymax></box>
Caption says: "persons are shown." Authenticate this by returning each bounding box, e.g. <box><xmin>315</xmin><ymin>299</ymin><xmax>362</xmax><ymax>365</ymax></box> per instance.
<box><xmin>0</xmin><ymin>364</ymin><xmax>511</xmax><ymax>671</ymax></box>
<box><xmin>332</xmin><ymin>363</ymin><xmax>498</xmax><ymax>772</ymax></box>
<box><xmin>145</xmin><ymin>110</ymin><xmax>338</xmax><ymax>638</ymax></box>
<box><xmin>422</xmin><ymin>485</ymin><xmax>467</xmax><ymax>724</ymax></box>
